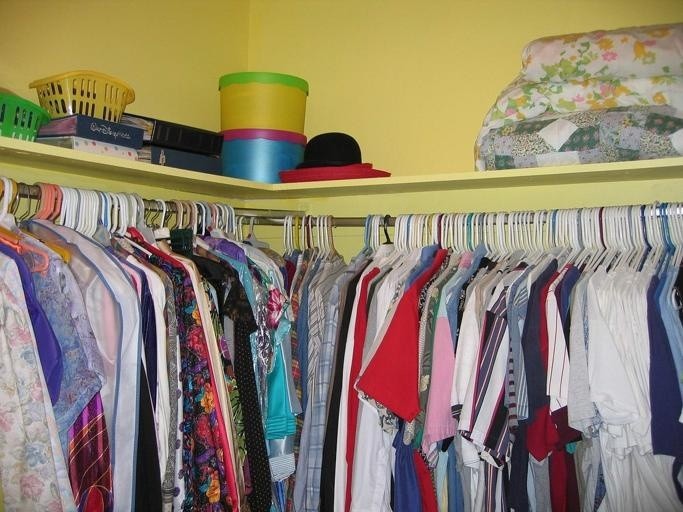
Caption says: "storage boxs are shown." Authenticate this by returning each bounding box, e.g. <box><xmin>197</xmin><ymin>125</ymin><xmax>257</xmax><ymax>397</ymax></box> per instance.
<box><xmin>219</xmin><ymin>72</ymin><xmax>309</xmax><ymax>183</ymax></box>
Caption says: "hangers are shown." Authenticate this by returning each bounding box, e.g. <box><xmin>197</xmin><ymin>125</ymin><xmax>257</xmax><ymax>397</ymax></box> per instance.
<box><xmin>0</xmin><ymin>176</ymin><xmax>270</xmax><ymax>249</ymax></box>
